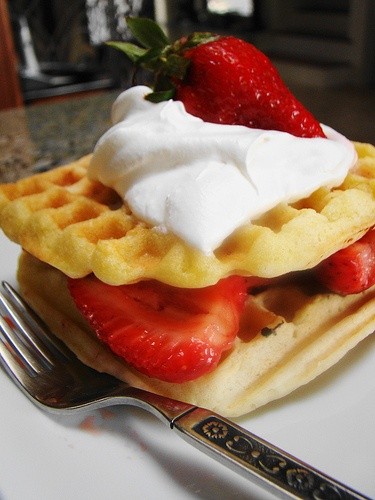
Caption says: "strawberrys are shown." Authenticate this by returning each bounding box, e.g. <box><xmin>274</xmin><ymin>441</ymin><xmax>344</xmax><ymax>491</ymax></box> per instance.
<box><xmin>100</xmin><ymin>13</ymin><xmax>327</xmax><ymax>138</ymax></box>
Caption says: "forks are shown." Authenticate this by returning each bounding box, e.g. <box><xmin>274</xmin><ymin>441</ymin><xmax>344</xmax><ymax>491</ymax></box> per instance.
<box><xmin>0</xmin><ymin>280</ymin><xmax>375</xmax><ymax>500</ymax></box>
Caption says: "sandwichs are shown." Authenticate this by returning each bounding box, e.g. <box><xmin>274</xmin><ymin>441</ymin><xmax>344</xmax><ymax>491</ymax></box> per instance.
<box><xmin>0</xmin><ymin>84</ymin><xmax>375</xmax><ymax>420</ymax></box>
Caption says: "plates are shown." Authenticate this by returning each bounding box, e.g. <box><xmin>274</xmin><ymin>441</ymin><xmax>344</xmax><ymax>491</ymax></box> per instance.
<box><xmin>0</xmin><ymin>229</ymin><xmax>375</xmax><ymax>500</ymax></box>
